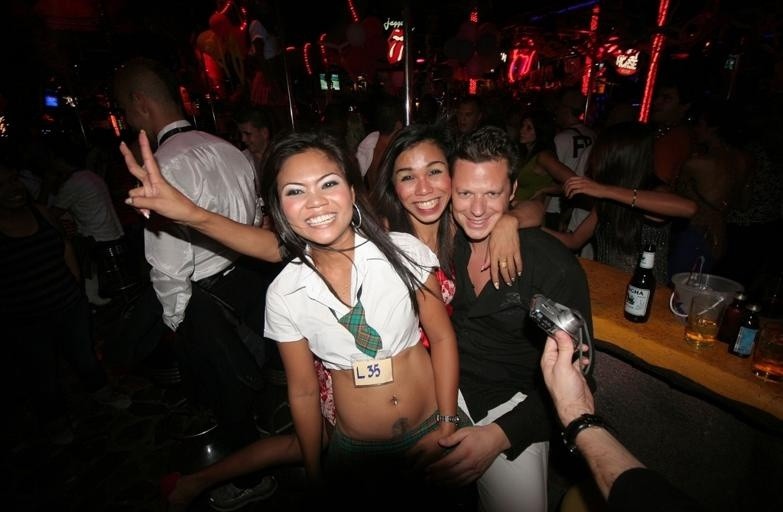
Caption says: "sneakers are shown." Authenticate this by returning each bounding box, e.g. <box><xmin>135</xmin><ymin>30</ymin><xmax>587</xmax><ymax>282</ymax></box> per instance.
<box><xmin>208</xmin><ymin>472</ymin><xmax>279</xmax><ymax>512</ymax></box>
<box><xmin>95</xmin><ymin>394</ymin><xmax>133</xmax><ymax>410</ymax></box>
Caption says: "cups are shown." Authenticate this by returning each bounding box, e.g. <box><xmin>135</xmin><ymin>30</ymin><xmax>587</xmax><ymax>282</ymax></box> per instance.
<box><xmin>686</xmin><ymin>296</ymin><xmax>725</xmax><ymax>349</ymax></box>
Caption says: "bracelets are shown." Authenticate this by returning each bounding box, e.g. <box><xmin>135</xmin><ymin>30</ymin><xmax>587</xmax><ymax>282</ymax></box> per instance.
<box><xmin>433</xmin><ymin>412</ymin><xmax>461</xmax><ymax>424</ymax></box>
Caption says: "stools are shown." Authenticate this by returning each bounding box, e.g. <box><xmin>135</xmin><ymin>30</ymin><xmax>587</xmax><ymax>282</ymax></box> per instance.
<box><xmin>96</xmin><ymin>236</ymin><xmax>145</xmax><ymax>322</ymax></box>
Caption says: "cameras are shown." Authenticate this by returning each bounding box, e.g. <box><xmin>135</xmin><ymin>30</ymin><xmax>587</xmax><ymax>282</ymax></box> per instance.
<box><xmin>526</xmin><ymin>293</ymin><xmax>584</xmax><ymax>350</ymax></box>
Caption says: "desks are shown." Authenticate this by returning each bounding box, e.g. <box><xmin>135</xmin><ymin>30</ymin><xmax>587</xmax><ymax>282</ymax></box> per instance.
<box><xmin>576</xmin><ymin>258</ymin><xmax>783</xmax><ymax>422</ymax></box>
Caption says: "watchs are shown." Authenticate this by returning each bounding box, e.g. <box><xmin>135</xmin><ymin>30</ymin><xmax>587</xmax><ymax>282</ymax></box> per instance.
<box><xmin>557</xmin><ymin>413</ymin><xmax>604</xmax><ymax>455</ymax></box>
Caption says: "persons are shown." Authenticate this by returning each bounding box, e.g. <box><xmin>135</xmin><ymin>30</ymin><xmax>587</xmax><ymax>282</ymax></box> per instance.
<box><xmin>177</xmin><ymin>0</ymin><xmax>517</xmax><ymax>132</ymax></box>
<box><xmin>121</xmin><ymin>121</ymin><xmax>546</xmax><ymax>512</ymax></box>
<box><xmin>76</xmin><ymin>135</ymin><xmax>115</xmax><ymax>174</ymax></box>
<box><xmin>263</xmin><ymin>133</ymin><xmax>460</xmax><ymax>511</ymax></box>
<box><xmin>422</xmin><ymin>124</ymin><xmax>592</xmax><ymax>511</ymax></box>
<box><xmin>538</xmin><ymin>328</ymin><xmax>703</xmax><ymax>511</ymax></box>
<box><xmin>29</xmin><ymin>145</ymin><xmax>125</xmax><ymax>307</ymax></box>
<box><xmin>108</xmin><ymin>63</ymin><xmax>258</xmax><ymax>427</ymax></box>
<box><xmin>231</xmin><ymin>109</ymin><xmax>273</xmax><ymax>231</ymax></box>
<box><xmin>0</xmin><ymin>160</ymin><xmax>132</xmax><ymax>446</ymax></box>
<box><xmin>517</xmin><ymin>0</ymin><xmax>782</xmax><ymax>291</ymax></box>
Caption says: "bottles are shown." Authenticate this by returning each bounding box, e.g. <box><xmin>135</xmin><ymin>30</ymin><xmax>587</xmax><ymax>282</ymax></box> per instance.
<box><xmin>717</xmin><ymin>292</ymin><xmax>747</xmax><ymax>344</ymax></box>
<box><xmin>624</xmin><ymin>244</ymin><xmax>656</xmax><ymax>323</ymax></box>
<box><xmin>728</xmin><ymin>304</ymin><xmax>761</xmax><ymax>359</ymax></box>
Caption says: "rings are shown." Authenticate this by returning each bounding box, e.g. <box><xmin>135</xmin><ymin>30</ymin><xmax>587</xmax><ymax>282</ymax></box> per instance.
<box><xmin>499</xmin><ymin>261</ymin><xmax>507</xmax><ymax>268</ymax></box>
